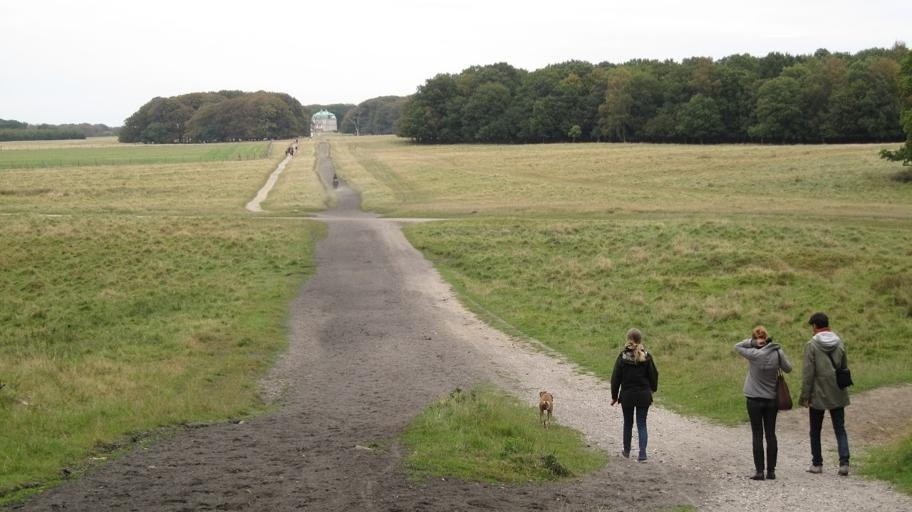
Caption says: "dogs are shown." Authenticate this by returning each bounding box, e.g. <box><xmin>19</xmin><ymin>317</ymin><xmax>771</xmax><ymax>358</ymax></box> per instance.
<box><xmin>536</xmin><ymin>388</ymin><xmax>553</xmax><ymax>422</ymax></box>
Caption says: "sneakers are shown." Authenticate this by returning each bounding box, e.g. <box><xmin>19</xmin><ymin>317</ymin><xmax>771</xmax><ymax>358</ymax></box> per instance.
<box><xmin>804</xmin><ymin>463</ymin><xmax>823</xmax><ymax>473</ymax></box>
<box><xmin>836</xmin><ymin>464</ymin><xmax>850</xmax><ymax>476</ymax></box>
<box><xmin>621</xmin><ymin>448</ymin><xmax>648</xmax><ymax>465</ymax></box>
<box><xmin>748</xmin><ymin>474</ymin><xmax>776</xmax><ymax>480</ymax></box>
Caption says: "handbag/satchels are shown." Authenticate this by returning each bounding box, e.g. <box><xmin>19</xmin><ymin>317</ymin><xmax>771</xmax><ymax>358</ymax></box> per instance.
<box><xmin>774</xmin><ymin>373</ymin><xmax>794</xmax><ymax>411</ymax></box>
<box><xmin>835</xmin><ymin>367</ymin><xmax>854</xmax><ymax>390</ymax></box>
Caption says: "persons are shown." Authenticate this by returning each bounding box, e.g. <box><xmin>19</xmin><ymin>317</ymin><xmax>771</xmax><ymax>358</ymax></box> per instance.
<box><xmin>610</xmin><ymin>327</ymin><xmax>658</xmax><ymax>463</ymax></box>
<box><xmin>798</xmin><ymin>312</ymin><xmax>851</xmax><ymax>476</ymax></box>
<box><xmin>734</xmin><ymin>325</ymin><xmax>792</xmax><ymax>480</ymax></box>
<box><xmin>285</xmin><ymin>145</ymin><xmax>297</xmax><ymax>157</ymax></box>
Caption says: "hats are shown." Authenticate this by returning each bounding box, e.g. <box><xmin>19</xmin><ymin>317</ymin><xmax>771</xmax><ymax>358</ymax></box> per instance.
<box><xmin>807</xmin><ymin>312</ymin><xmax>829</xmax><ymax>324</ymax></box>
